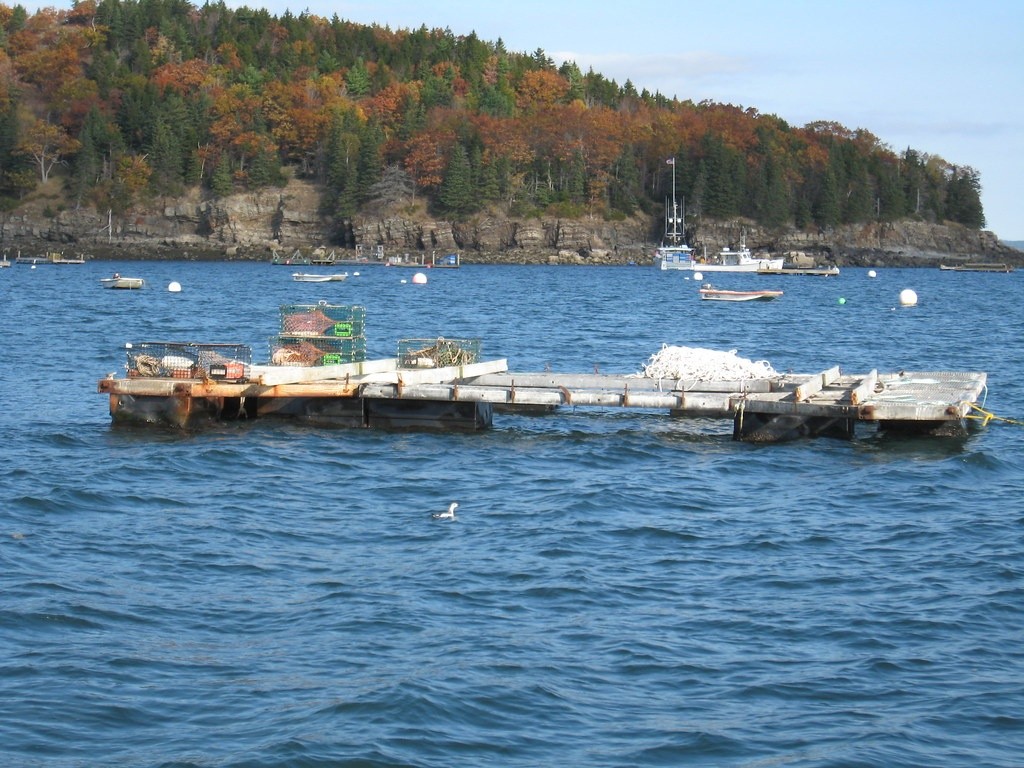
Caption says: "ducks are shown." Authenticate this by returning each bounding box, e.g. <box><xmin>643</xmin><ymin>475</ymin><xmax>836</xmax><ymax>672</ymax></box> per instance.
<box><xmin>430</xmin><ymin>502</ymin><xmax>461</xmax><ymax>519</ymax></box>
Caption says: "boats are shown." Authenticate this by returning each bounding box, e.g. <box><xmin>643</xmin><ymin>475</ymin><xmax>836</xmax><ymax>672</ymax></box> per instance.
<box><xmin>694</xmin><ymin>233</ymin><xmax>784</xmax><ymax>273</ymax></box>
<box><xmin>700</xmin><ymin>284</ymin><xmax>783</xmax><ymax>303</ymax></box>
<box><xmin>100</xmin><ymin>275</ymin><xmax>146</xmax><ymax>289</ymax></box>
<box><xmin>954</xmin><ymin>261</ymin><xmax>1014</xmax><ymax>274</ymax></box>
<box><xmin>938</xmin><ymin>264</ymin><xmax>958</xmax><ymax>270</ymax></box>
<box><xmin>292</xmin><ymin>271</ymin><xmax>349</xmax><ymax>282</ymax></box>
<box><xmin>655</xmin><ymin>159</ymin><xmax>697</xmax><ymax>271</ymax></box>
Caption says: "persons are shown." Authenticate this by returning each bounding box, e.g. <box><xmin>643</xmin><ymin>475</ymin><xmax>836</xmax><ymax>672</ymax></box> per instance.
<box><xmin>691</xmin><ymin>250</ymin><xmax>721</xmax><ymax>265</ymax></box>
<box><xmin>113</xmin><ymin>274</ymin><xmax>116</xmax><ymax>279</ymax></box>
